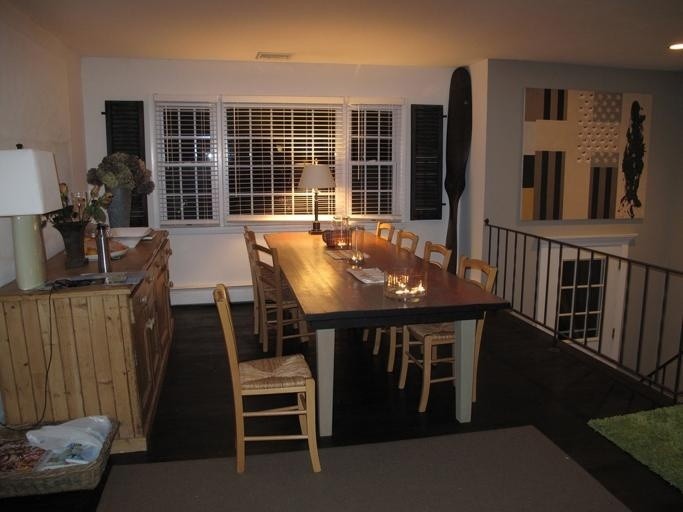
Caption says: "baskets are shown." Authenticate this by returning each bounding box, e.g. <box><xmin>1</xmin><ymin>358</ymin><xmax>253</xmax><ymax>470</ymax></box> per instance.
<box><xmin>1</xmin><ymin>415</ymin><xmax>120</xmax><ymax>499</ymax></box>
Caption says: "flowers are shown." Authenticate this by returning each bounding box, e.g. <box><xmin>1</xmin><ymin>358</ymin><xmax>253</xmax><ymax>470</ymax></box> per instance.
<box><xmin>50</xmin><ymin>182</ymin><xmax>113</xmax><ymax>224</ymax></box>
<box><xmin>86</xmin><ymin>150</ymin><xmax>156</xmax><ymax>201</ymax></box>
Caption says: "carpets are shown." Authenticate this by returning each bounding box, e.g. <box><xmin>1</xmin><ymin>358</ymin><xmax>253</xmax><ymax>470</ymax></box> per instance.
<box><xmin>95</xmin><ymin>426</ymin><xmax>632</xmax><ymax>511</ymax></box>
<box><xmin>588</xmin><ymin>404</ymin><xmax>682</xmax><ymax>496</ymax></box>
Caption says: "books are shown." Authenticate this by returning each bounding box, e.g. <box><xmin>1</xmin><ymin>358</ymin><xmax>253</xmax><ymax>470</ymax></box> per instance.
<box><xmin>34</xmin><ymin>442</ymin><xmax>96</xmax><ymax>469</ymax></box>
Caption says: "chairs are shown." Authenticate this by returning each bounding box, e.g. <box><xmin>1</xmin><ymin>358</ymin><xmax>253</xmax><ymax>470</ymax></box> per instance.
<box><xmin>212</xmin><ymin>281</ymin><xmax>324</xmax><ymax>475</ymax></box>
<box><xmin>238</xmin><ymin>223</ymin><xmax>315</xmax><ymax>357</ymax></box>
<box><xmin>363</xmin><ymin>219</ymin><xmax>500</xmax><ymax>416</ymax></box>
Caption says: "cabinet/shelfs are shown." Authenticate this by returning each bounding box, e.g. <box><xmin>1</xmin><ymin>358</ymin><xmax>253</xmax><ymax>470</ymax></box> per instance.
<box><xmin>1</xmin><ymin>224</ymin><xmax>179</xmax><ymax>455</ymax></box>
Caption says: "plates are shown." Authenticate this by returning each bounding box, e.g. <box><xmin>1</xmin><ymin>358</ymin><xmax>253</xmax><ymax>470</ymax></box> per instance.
<box><xmin>85</xmin><ymin>245</ymin><xmax>129</xmax><ymax>259</ymax></box>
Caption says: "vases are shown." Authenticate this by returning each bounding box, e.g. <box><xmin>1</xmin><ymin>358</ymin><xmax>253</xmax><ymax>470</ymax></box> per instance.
<box><xmin>54</xmin><ymin>222</ymin><xmax>92</xmax><ymax>270</ymax></box>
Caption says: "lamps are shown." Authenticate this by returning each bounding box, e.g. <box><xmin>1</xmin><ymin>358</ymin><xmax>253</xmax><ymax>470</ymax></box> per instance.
<box><xmin>0</xmin><ymin>142</ymin><xmax>66</xmax><ymax>291</ymax></box>
<box><xmin>296</xmin><ymin>160</ymin><xmax>337</xmax><ymax>237</ymax></box>
